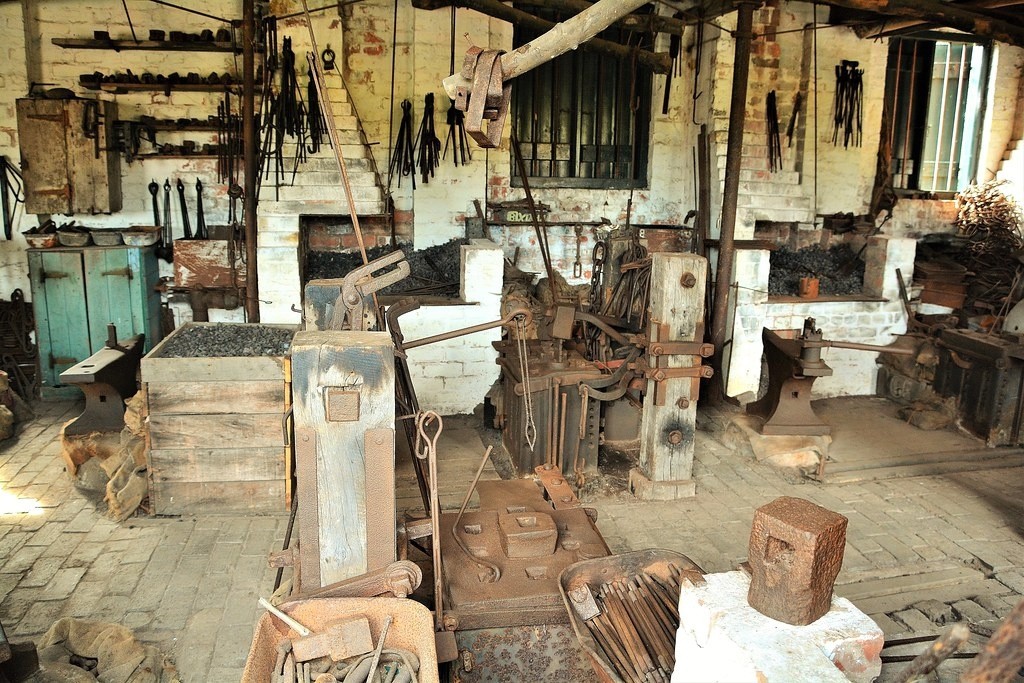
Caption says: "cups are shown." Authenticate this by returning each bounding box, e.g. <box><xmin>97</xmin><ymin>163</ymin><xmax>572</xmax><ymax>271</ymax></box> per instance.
<box><xmin>798</xmin><ymin>277</ymin><xmax>819</xmax><ymax>298</ymax></box>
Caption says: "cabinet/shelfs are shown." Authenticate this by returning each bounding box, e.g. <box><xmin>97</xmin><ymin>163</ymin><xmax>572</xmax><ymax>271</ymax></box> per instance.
<box><xmin>16</xmin><ymin>98</ymin><xmax>123</xmax><ymax>217</ymax></box>
<box><xmin>25</xmin><ymin>245</ymin><xmax>160</xmax><ymax>399</ymax></box>
<box><xmin>51</xmin><ymin>37</ymin><xmax>271</xmax><ymax>160</ymax></box>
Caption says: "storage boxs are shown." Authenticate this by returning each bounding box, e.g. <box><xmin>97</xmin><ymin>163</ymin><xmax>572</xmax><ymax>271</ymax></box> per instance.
<box><xmin>141</xmin><ymin>323</ymin><xmax>294</xmax><ymax>518</ymax></box>
